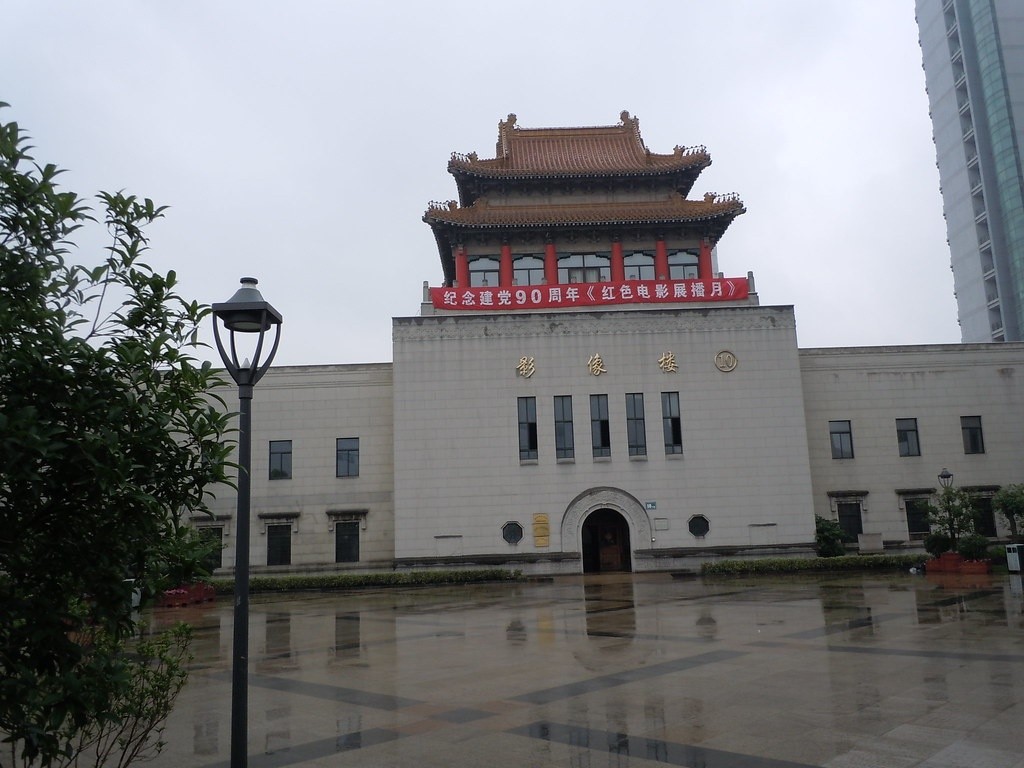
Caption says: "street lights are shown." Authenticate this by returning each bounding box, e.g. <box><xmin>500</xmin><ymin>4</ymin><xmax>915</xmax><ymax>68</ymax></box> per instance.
<box><xmin>938</xmin><ymin>467</ymin><xmax>955</xmax><ymax>539</ymax></box>
<box><xmin>213</xmin><ymin>279</ymin><xmax>282</xmax><ymax>768</ymax></box>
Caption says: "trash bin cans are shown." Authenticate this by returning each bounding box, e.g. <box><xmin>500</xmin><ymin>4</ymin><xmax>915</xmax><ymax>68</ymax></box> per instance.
<box><xmin>1005</xmin><ymin>544</ymin><xmax>1024</xmax><ymax>574</ymax></box>
